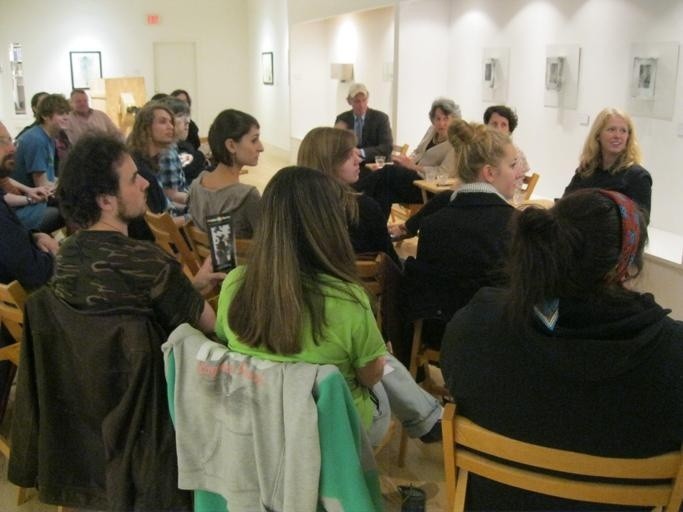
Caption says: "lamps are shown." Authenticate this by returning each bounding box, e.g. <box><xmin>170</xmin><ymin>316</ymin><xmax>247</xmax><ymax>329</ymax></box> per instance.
<box><xmin>331</xmin><ymin>62</ymin><xmax>355</xmax><ymax>82</ymax></box>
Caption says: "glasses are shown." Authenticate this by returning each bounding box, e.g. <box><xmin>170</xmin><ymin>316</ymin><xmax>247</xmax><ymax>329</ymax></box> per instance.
<box><xmin>0</xmin><ymin>139</ymin><xmax>22</xmax><ymax>150</ymax></box>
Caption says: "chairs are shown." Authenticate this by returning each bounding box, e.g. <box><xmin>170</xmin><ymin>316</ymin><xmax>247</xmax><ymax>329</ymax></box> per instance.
<box><xmin>183</xmin><ymin>223</ymin><xmax>258</xmax><ymax>292</ymax></box>
<box><xmin>0</xmin><ymin>283</ymin><xmax>35</xmax><ymax>505</ymax></box>
<box><xmin>441</xmin><ymin>401</ymin><xmax>682</xmax><ymax>511</ymax></box>
<box><xmin>390</xmin><ymin>144</ymin><xmax>409</xmax><ymax>158</ymax></box>
<box><xmin>355</xmin><ymin>253</ymin><xmax>385</xmax><ymax>331</ymax></box>
<box><xmin>391</xmin><ymin>207</ymin><xmax>420</xmax><ymax>247</ymax></box>
<box><xmin>143</xmin><ymin>210</ymin><xmax>198</xmax><ymax>277</ymax></box>
<box><xmin>396</xmin><ymin>316</ymin><xmax>439</xmax><ymax>466</ymax></box>
<box><xmin>26</xmin><ymin>294</ymin><xmax>168</xmax><ymax>512</ymax></box>
<box><xmin>515</xmin><ymin>173</ymin><xmax>540</xmax><ymax>203</ymax></box>
<box><xmin>172</xmin><ymin>324</ymin><xmax>399</xmax><ymax>512</ymax></box>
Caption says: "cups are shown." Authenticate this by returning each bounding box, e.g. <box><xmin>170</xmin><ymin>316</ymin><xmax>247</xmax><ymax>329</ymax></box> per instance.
<box><xmin>425</xmin><ymin>165</ymin><xmax>436</xmax><ymax>183</ymax></box>
<box><xmin>438</xmin><ymin>172</ymin><xmax>450</xmax><ymax>185</ymax></box>
<box><xmin>375</xmin><ymin>156</ymin><xmax>385</xmax><ymax>168</ymax></box>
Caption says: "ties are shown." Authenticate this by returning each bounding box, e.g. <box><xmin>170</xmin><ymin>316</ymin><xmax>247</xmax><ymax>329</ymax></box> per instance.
<box><xmin>355</xmin><ymin>116</ymin><xmax>362</xmax><ymax>148</ymax></box>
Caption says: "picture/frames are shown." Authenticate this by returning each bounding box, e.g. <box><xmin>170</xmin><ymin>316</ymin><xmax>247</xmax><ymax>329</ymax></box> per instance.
<box><xmin>70</xmin><ymin>51</ymin><xmax>102</xmax><ymax>91</ymax></box>
<box><xmin>262</xmin><ymin>52</ymin><xmax>273</xmax><ymax>85</ymax></box>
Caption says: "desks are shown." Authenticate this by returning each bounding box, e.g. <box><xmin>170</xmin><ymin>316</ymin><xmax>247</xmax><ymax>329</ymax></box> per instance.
<box><xmin>413</xmin><ymin>179</ymin><xmax>461</xmax><ymax>202</ymax></box>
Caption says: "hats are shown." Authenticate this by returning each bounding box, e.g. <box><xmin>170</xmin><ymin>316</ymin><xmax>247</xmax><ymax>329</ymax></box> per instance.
<box><xmin>348</xmin><ymin>84</ymin><xmax>369</xmax><ymax>98</ymax></box>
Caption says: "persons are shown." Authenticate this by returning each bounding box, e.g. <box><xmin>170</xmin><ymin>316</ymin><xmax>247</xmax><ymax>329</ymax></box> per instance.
<box><xmin>0</xmin><ymin>82</ymin><xmax>530</xmax><ymax>512</ymax></box>
<box><xmin>440</xmin><ymin>189</ymin><xmax>683</xmax><ymax>512</ymax></box>
<box><xmin>562</xmin><ymin>108</ymin><xmax>652</xmax><ymax>227</ymax></box>
